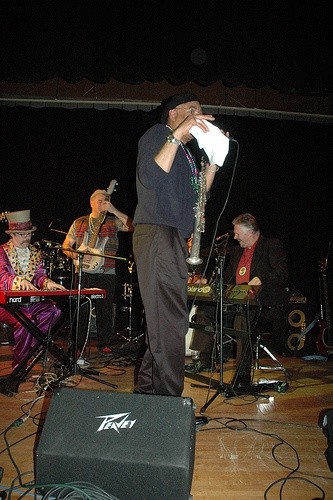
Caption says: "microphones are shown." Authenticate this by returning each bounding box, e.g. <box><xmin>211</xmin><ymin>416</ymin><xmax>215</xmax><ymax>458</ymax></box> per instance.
<box><xmin>36</xmin><ymin>242</ymin><xmax>51</xmax><ymax>246</ymax></box>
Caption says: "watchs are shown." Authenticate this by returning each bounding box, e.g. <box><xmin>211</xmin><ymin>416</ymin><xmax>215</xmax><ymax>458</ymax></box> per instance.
<box><xmin>167</xmin><ymin>135</ymin><xmax>180</xmax><ymax>144</ymax></box>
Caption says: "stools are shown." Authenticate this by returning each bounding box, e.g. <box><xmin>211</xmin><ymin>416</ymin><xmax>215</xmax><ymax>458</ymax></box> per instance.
<box><xmin>0</xmin><ymin>323</ymin><xmax>15</xmax><ymax>347</ymax></box>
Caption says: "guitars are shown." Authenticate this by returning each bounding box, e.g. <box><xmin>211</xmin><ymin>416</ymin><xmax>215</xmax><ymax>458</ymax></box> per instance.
<box><xmin>315</xmin><ymin>256</ymin><xmax>333</xmax><ymax>358</ymax></box>
<box><xmin>73</xmin><ymin>178</ymin><xmax>120</xmax><ymax>274</ymax></box>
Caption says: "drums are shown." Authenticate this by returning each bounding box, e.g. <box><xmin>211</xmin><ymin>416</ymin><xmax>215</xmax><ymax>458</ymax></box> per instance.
<box><xmin>81</xmin><ymin>302</ymin><xmax>119</xmax><ymax>337</ymax></box>
<box><xmin>43</xmin><ymin>258</ymin><xmax>76</xmax><ymax>288</ymax></box>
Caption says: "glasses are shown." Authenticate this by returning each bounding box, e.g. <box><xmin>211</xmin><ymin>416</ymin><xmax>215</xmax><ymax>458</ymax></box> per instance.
<box><xmin>172</xmin><ymin>107</ymin><xmax>199</xmax><ymax>114</ymax></box>
<box><xmin>17</xmin><ymin>232</ymin><xmax>35</xmax><ymax>237</ymax></box>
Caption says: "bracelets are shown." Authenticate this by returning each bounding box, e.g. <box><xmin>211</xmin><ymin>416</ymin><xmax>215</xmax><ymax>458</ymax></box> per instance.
<box><xmin>206</xmin><ymin>166</ymin><xmax>219</xmax><ymax>172</ymax></box>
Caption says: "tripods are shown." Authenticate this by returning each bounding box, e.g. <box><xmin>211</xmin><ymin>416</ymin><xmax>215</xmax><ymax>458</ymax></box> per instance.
<box><xmin>36</xmin><ymin>246</ymin><xmax>128</xmax><ymax>396</ymax></box>
<box><xmin>190</xmin><ymin>233</ymin><xmax>290</xmax><ymax>412</ymax></box>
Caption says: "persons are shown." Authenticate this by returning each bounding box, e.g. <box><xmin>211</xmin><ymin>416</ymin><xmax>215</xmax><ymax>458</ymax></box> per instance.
<box><xmin>184</xmin><ymin>213</ymin><xmax>287</xmax><ymax>387</ymax></box>
<box><xmin>0</xmin><ymin>210</ymin><xmax>66</xmax><ymax>371</ymax></box>
<box><xmin>132</xmin><ymin>93</ymin><xmax>229</xmax><ymax>428</ymax></box>
<box><xmin>63</xmin><ymin>189</ymin><xmax>134</xmax><ymax>356</ymax></box>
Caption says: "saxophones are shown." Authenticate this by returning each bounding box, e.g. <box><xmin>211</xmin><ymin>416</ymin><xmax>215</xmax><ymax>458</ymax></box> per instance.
<box><xmin>185</xmin><ymin>154</ymin><xmax>206</xmax><ymax>265</ymax></box>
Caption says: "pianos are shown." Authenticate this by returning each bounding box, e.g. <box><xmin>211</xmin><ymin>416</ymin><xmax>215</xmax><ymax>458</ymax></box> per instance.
<box><xmin>185</xmin><ymin>281</ymin><xmax>289</xmax><ymax>412</ymax></box>
<box><xmin>0</xmin><ymin>286</ymin><xmax>107</xmax><ymax>395</ymax></box>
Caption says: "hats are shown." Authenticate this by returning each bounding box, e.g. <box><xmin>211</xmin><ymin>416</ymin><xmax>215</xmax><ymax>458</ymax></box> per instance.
<box><xmin>161</xmin><ymin>92</ymin><xmax>200</xmax><ymax>125</ymax></box>
<box><xmin>5</xmin><ymin>210</ymin><xmax>36</xmax><ymax>234</ymax></box>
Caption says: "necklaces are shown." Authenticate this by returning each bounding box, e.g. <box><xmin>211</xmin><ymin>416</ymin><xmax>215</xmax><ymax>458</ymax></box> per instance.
<box><xmin>239</xmin><ymin>256</ymin><xmax>249</xmax><ymax>276</ymax></box>
<box><xmin>166</xmin><ymin>125</ymin><xmax>197</xmax><ymax>192</ymax></box>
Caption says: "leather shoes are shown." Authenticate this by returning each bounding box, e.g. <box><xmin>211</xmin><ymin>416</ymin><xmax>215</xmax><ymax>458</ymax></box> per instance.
<box><xmin>182</xmin><ymin>360</ymin><xmax>215</xmax><ymax>373</ymax></box>
<box><xmin>236</xmin><ymin>380</ymin><xmax>250</xmax><ymax>390</ymax></box>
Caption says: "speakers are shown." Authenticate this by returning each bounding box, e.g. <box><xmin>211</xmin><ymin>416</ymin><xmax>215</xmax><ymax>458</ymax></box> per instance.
<box><xmin>35</xmin><ymin>387</ymin><xmax>196</xmax><ymax>500</ymax></box>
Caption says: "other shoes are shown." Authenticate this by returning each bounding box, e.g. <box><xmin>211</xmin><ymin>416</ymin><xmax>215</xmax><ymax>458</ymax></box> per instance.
<box><xmin>99</xmin><ymin>346</ymin><xmax>113</xmax><ymax>356</ymax></box>
<box><xmin>30</xmin><ymin>348</ymin><xmax>47</xmax><ymax>363</ymax></box>
<box><xmin>194</xmin><ymin>416</ymin><xmax>207</xmax><ymax>427</ymax></box>
